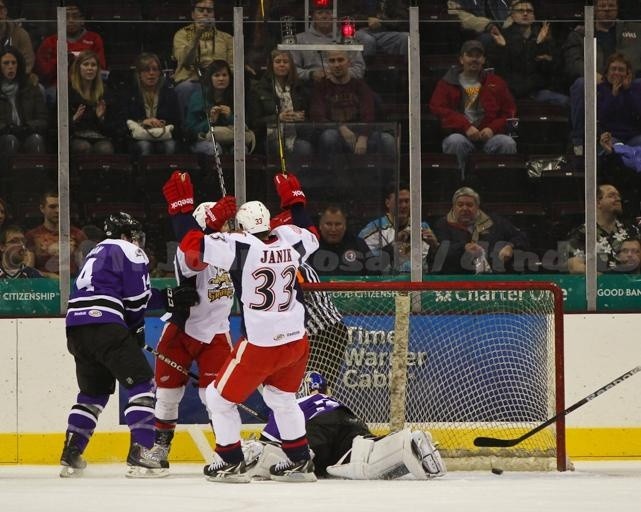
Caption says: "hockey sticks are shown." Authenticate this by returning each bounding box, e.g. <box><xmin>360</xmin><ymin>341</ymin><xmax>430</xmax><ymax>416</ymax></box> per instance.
<box><xmin>140</xmin><ymin>342</ymin><xmax>269</xmax><ymax>422</ymax></box>
<box><xmin>474</xmin><ymin>366</ymin><xmax>641</xmax><ymax>447</ymax></box>
<box><xmin>196</xmin><ymin>65</ymin><xmax>236</xmax><ymax>232</ymax></box>
<box><xmin>260</xmin><ymin>1</ymin><xmax>287</xmax><ymax>173</ymax></box>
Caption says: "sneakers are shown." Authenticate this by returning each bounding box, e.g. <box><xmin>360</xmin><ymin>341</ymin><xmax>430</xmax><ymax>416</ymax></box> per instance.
<box><xmin>155</xmin><ymin>430</ymin><xmax>173</xmax><ymax>456</ymax></box>
<box><xmin>204</xmin><ymin>444</ymin><xmax>247</xmax><ymax>475</ymax></box>
<box><xmin>269</xmin><ymin>447</ymin><xmax>316</xmax><ymax>476</ymax></box>
<box><xmin>410</xmin><ymin>429</ymin><xmax>448</xmax><ymax>479</ymax></box>
<box><xmin>126</xmin><ymin>445</ymin><xmax>170</xmax><ymax>469</ymax></box>
<box><xmin>59</xmin><ymin>444</ymin><xmax>88</xmax><ymax>468</ymax></box>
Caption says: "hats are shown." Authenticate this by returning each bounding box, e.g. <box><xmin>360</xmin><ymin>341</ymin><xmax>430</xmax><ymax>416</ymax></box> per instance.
<box><xmin>459</xmin><ymin>41</ymin><xmax>486</xmax><ymax>56</ymax></box>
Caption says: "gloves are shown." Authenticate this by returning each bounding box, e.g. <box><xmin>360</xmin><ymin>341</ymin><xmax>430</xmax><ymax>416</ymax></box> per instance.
<box><xmin>205</xmin><ymin>197</ymin><xmax>236</xmax><ymax>232</ymax></box>
<box><xmin>274</xmin><ymin>173</ymin><xmax>306</xmax><ymax>209</ymax></box>
<box><xmin>163</xmin><ymin>170</ymin><xmax>193</xmax><ymax>216</ymax></box>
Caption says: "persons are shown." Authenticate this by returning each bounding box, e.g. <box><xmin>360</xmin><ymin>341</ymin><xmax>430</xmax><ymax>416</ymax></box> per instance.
<box><xmin>295</xmin><ymin>262</ymin><xmax>351</xmax><ymax>397</ymax></box>
<box><xmin>0</xmin><ymin>45</ymin><xmax>51</xmax><ymax>172</ymax></box>
<box><xmin>151</xmin><ymin>194</ymin><xmax>294</xmax><ymax>476</ymax></box>
<box><xmin>67</xmin><ymin>48</ymin><xmax>117</xmax><ymax>164</ymax></box>
<box><xmin>24</xmin><ymin>191</ymin><xmax>88</xmax><ymax>278</ymax></box>
<box><xmin>562</xmin><ymin>0</ymin><xmax>641</xmax><ymax>164</ymax></box>
<box><xmin>618</xmin><ymin>238</ymin><xmax>640</xmax><ymax>273</ymax></box>
<box><xmin>429</xmin><ymin>40</ymin><xmax>519</xmax><ymax>182</ymax></box>
<box><xmin>492</xmin><ymin>2</ymin><xmax>560</xmax><ymax>98</ymax></box>
<box><xmin>0</xmin><ymin>0</ymin><xmax>46</xmax><ymax>103</ymax></box>
<box><xmin>360</xmin><ymin>184</ymin><xmax>442</xmax><ymax>276</ymax></box>
<box><xmin>595</xmin><ymin>53</ymin><xmax>641</xmax><ymax>148</ymax></box>
<box><xmin>306</xmin><ymin>204</ymin><xmax>380</xmax><ymax>275</ymax></box>
<box><xmin>159</xmin><ymin>168</ymin><xmax>323</xmax><ymax>475</ymax></box>
<box><xmin>0</xmin><ymin>201</ymin><xmax>7</xmax><ymax>224</ymax></box>
<box><xmin>556</xmin><ymin>183</ymin><xmax>641</xmax><ymax>274</ymax></box>
<box><xmin>309</xmin><ymin>48</ymin><xmax>402</xmax><ymax>191</ymax></box>
<box><xmin>245</xmin><ymin>46</ymin><xmax>315</xmax><ymax>168</ymax></box>
<box><xmin>240</xmin><ymin>370</ymin><xmax>448</xmax><ymax>480</ymax></box>
<box><xmin>183</xmin><ymin>59</ymin><xmax>255</xmax><ymax>156</ymax></box>
<box><xmin>170</xmin><ymin>0</ymin><xmax>254</xmax><ymax>84</ymax></box>
<box><xmin>0</xmin><ymin>224</ymin><xmax>44</xmax><ymax>279</ymax></box>
<box><xmin>110</xmin><ymin>52</ymin><xmax>182</xmax><ymax>159</ymax></box>
<box><xmin>598</xmin><ymin>130</ymin><xmax>641</xmax><ymax>196</ymax></box>
<box><xmin>430</xmin><ymin>187</ymin><xmax>527</xmax><ymax>274</ymax></box>
<box><xmin>283</xmin><ymin>1</ymin><xmax>367</xmax><ymax>82</ymax></box>
<box><xmin>355</xmin><ymin>1</ymin><xmax>409</xmax><ymax>81</ymax></box>
<box><xmin>60</xmin><ymin>211</ymin><xmax>172</xmax><ymax>471</ymax></box>
<box><xmin>36</xmin><ymin>0</ymin><xmax>108</xmax><ymax>85</ymax></box>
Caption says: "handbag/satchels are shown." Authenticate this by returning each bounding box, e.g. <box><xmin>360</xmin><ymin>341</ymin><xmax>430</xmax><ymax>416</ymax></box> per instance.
<box><xmin>126</xmin><ymin>120</ymin><xmax>174</xmax><ymax>139</ymax></box>
<box><xmin>198</xmin><ymin>126</ymin><xmax>256</xmax><ymax>155</ymax></box>
<box><xmin>71</xmin><ymin>128</ymin><xmax>105</xmax><ymax>140</ymax></box>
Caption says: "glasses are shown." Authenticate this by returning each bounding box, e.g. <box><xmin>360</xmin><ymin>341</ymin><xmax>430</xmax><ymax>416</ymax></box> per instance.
<box><xmin>194</xmin><ymin>4</ymin><xmax>215</xmax><ymax>15</ymax></box>
<box><xmin>2</xmin><ymin>237</ymin><xmax>28</xmax><ymax>245</ymax></box>
<box><xmin>594</xmin><ymin>7</ymin><xmax>620</xmax><ymax>15</ymax></box>
<box><xmin>512</xmin><ymin>7</ymin><xmax>536</xmax><ymax>13</ymax></box>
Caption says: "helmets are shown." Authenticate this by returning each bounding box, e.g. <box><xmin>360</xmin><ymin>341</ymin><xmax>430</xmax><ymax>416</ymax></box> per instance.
<box><xmin>103</xmin><ymin>212</ymin><xmax>142</xmax><ymax>240</ymax></box>
<box><xmin>235</xmin><ymin>200</ymin><xmax>272</xmax><ymax>236</ymax></box>
<box><xmin>192</xmin><ymin>200</ymin><xmax>218</xmax><ymax>233</ymax></box>
<box><xmin>297</xmin><ymin>370</ymin><xmax>328</xmax><ymax>398</ymax></box>
